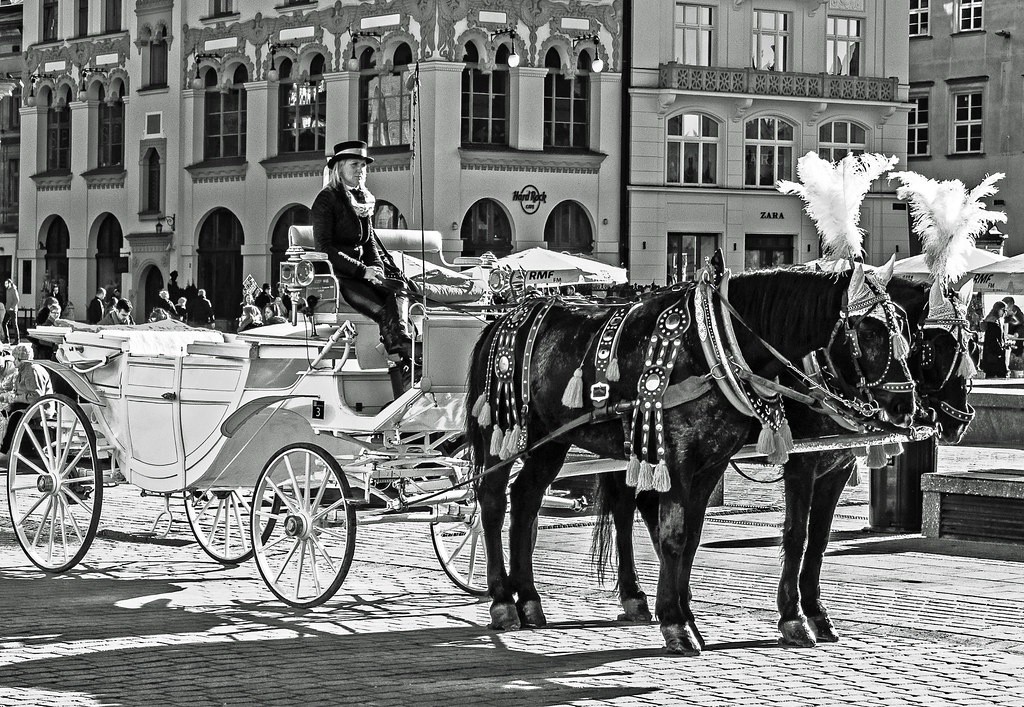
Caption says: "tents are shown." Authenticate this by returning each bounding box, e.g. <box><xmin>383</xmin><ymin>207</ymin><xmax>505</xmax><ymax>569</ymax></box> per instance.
<box><xmin>457</xmin><ymin>247</ymin><xmax>627</xmax><ymax>285</ymax></box>
<box><xmin>799</xmin><ymin>249</ymin><xmax>1024</xmax><ymax>294</ymax></box>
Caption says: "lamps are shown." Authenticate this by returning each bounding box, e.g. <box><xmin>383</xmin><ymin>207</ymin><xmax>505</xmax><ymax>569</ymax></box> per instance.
<box><xmin>193</xmin><ymin>53</ymin><xmax>221</xmax><ymax>90</ymax></box>
<box><xmin>572</xmin><ymin>33</ymin><xmax>603</xmax><ymax>73</ymax></box>
<box><xmin>155</xmin><ymin>213</ymin><xmax>175</xmax><ymax>235</ymax></box>
<box><xmin>28</xmin><ymin>73</ymin><xmax>54</xmax><ymax>107</ymax></box>
<box><xmin>268</xmin><ymin>42</ymin><xmax>297</xmax><ymax>83</ymax></box>
<box><xmin>490</xmin><ymin>27</ymin><xmax>520</xmax><ymax>68</ymax></box>
<box><xmin>79</xmin><ymin>67</ymin><xmax>108</xmax><ymax>102</ymax></box>
<box><xmin>348</xmin><ymin>31</ymin><xmax>381</xmax><ymax>72</ymax></box>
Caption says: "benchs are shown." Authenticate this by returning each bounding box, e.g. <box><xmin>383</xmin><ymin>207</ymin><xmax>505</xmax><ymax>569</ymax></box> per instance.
<box><xmin>286</xmin><ymin>225</ymin><xmax>484</xmax><ymax>304</ymax></box>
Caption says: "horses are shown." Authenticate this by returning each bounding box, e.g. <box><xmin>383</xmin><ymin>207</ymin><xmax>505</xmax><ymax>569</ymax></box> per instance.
<box><xmin>462</xmin><ymin>253</ymin><xmax>974</xmax><ymax>657</ymax></box>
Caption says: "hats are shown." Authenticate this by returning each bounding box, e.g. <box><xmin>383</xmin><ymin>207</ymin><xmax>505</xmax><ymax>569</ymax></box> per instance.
<box><xmin>12</xmin><ymin>345</ymin><xmax>34</xmax><ymax>361</ymax></box>
<box><xmin>327</xmin><ymin>140</ymin><xmax>375</xmax><ymax>170</ymax></box>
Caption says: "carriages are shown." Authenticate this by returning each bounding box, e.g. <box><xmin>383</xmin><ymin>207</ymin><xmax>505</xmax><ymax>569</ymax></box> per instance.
<box><xmin>7</xmin><ymin>224</ymin><xmax>976</xmax><ymax>657</ymax></box>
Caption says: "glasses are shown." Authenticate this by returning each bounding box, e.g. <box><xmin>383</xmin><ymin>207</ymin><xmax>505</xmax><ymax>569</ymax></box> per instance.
<box><xmin>52</xmin><ymin>311</ymin><xmax>59</xmax><ymax>314</ymax></box>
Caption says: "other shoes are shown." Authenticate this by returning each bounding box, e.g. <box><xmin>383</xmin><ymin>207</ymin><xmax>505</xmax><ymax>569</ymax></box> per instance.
<box><xmin>10</xmin><ymin>340</ymin><xmax>19</xmax><ymax>345</ymax></box>
<box><xmin>2</xmin><ymin>339</ymin><xmax>9</xmax><ymax>344</ymax></box>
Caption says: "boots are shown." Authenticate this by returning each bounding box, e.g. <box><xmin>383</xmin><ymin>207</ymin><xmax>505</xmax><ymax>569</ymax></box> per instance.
<box><xmin>380</xmin><ymin>326</ymin><xmax>412</xmax><ymax>355</ymax></box>
<box><xmin>387</xmin><ymin>366</ymin><xmax>404</xmax><ymax>401</ymax></box>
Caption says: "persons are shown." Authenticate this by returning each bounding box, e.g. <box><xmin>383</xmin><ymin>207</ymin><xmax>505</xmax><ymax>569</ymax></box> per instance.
<box><xmin>0</xmin><ymin>345</ymin><xmax>55</xmax><ymax>455</ymax></box>
<box><xmin>980</xmin><ymin>297</ymin><xmax>1014</xmax><ymax>379</ymax></box>
<box><xmin>148</xmin><ymin>289</ymin><xmax>216</xmax><ymax>329</ymax></box>
<box><xmin>2</xmin><ymin>279</ymin><xmax>20</xmax><ymax>346</ymax></box>
<box><xmin>312</xmin><ymin>141</ymin><xmax>423</xmax><ymax>386</ymax></box>
<box><xmin>238</xmin><ymin>282</ymin><xmax>292</xmax><ymax>332</ymax></box>
<box><xmin>36</xmin><ymin>297</ymin><xmax>61</xmax><ymax>326</ymax></box>
<box><xmin>87</xmin><ymin>279</ymin><xmax>136</xmax><ymax>325</ymax></box>
